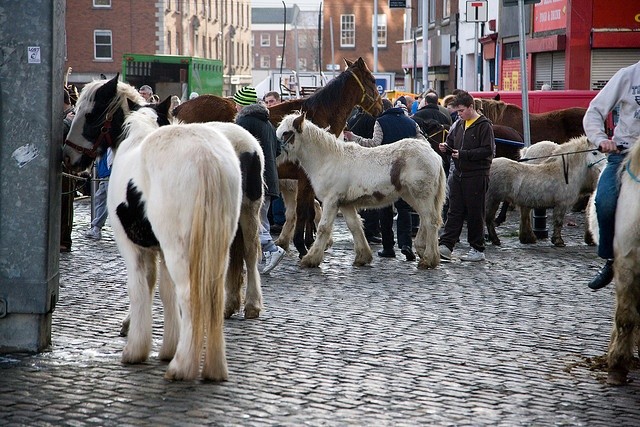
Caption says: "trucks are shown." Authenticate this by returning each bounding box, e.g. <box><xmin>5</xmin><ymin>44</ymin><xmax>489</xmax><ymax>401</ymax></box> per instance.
<box><xmin>467</xmin><ymin>90</ymin><xmax>614</xmax><ymax>141</ymax></box>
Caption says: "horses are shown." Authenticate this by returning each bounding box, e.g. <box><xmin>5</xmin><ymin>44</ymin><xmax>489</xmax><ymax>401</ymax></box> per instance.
<box><xmin>104</xmin><ymin>93</ymin><xmax>243</xmax><ymax>384</ymax></box>
<box><xmin>585</xmin><ymin>138</ymin><xmax>640</xmax><ymax>385</ymax></box>
<box><xmin>59</xmin><ymin>72</ymin><xmax>267</xmax><ymax>321</ymax></box>
<box><xmin>444</xmin><ymin>89</ymin><xmax>611</xmax><ymax>145</ymax></box>
<box><xmin>274</xmin><ymin>109</ymin><xmax>447</xmax><ymax>270</ymax></box>
<box><xmin>484</xmin><ymin>134</ymin><xmax>608</xmax><ymax>248</ymax></box>
<box><xmin>166</xmin><ymin>56</ymin><xmax>384</xmax><ymax>261</ymax></box>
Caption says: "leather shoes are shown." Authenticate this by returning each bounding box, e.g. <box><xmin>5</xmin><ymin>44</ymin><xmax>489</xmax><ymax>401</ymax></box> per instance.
<box><xmin>378</xmin><ymin>248</ymin><xmax>396</xmax><ymax>257</ymax></box>
<box><xmin>365</xmin><ymin>235</ymin><xmax>382</xmax><ymax>243</ymax></box>
<box><xmin>401</xmin><ymin>245</ymin><xmax>416</xmax><ymax>260</ymax></box>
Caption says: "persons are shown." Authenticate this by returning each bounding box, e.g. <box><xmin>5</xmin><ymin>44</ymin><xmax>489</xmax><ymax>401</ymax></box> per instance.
<box><xmin>443</xmin><ymin>94</ymin><xmax>460</xmax><ymax>121</ymax></box>
<box><xmin>344</xmin><ymin>98</ymin><xmax>422</xmax><ymax>258</ymax></box>
<box><xmin>582</xmin><ymin>61</ymin><xmax>640</xmax><ymax>290</ymax></box>
<box><xmin>84</xmin><ymin>81</ymin><xmax>162</xmax><ymax>242</ymax></box>
<box><xmin>163</xmin><ymin>92</ymin><xmax>202</xmax><ymax>125</ymax></box>
<box><xmin>437</xmin><ymin>92</ymin><xmax>493</xmax><ymax>262</ymax></box>
<box><xmin>62</xmin><ymin>88</ymin><xmax>78</xmax><ymax>252</ymax></box>
<box><xmin>233</xmin><ymin>86</ymin><xmax>287</xmax><ymax>275</ymax></box>
<box><xmin>412</xmin><ymin>91</ymin><xmax>453</xmax><ymax>151</ymax></box>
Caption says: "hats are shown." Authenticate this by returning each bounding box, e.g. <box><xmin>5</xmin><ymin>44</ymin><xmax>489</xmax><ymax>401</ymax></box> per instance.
<box><xmin>233</xmin><ymin>86</ymin><xmax>257</xmax><ymax>106</ymax></box>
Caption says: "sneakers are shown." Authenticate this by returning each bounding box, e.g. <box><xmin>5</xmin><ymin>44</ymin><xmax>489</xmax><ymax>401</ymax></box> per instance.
<box><xmin>262</xmin><ymin>245</ymin><xmax>286</xmax><ymax>274</ymax></box>
<box><xmin>438</xmin><ymin>245</ymin><xmax>451</xmax><ymax>260</ymax></box>
<box><xmin>588</xmin><ymin>264</ymin><xmax>614</xmax><ymax>289</ymax></box>
<box><xmin>79</xmin><ymin>228</ymin><xmax>102</xmax><ymax>240</ymax></box>
<box><xmin>257</xmin><ymin>263</ymin><xmax>271</xmax><ymax>274</ymax></box>
<box><xmin>460</xmin><ymin>246</ymin><xmax>485</xmax><ymax>261</ymax></box>
